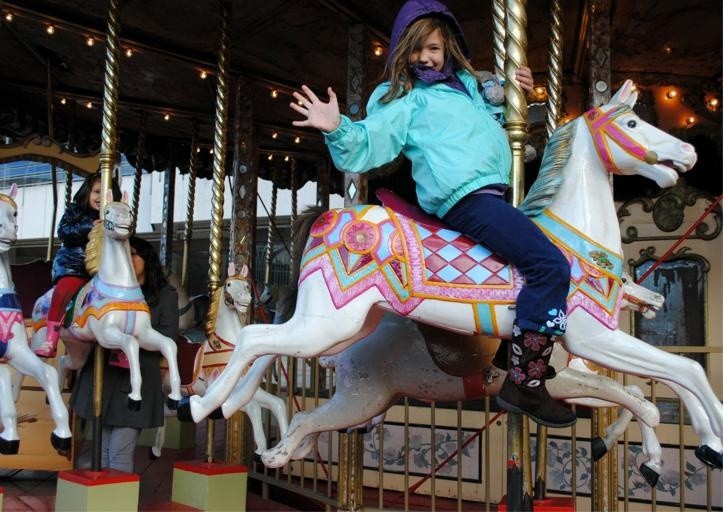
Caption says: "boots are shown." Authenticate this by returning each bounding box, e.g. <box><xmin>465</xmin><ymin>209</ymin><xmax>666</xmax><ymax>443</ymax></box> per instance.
<box><xmin>489</xmin><ymin>316</ymin><xmax>578</xmax><ymax>428</ymax></box>
<box><xmin>33</xmin><ymin>313</ymin><xmax>66</xmax><ymax>359</ymax></box>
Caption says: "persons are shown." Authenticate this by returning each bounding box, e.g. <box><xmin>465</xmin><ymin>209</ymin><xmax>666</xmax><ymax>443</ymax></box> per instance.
<box><xmin>69</xmin><ymin>235</ymin><xmax>178</xmax><ymax>474</ymax></box>
<box><xmin>35</xmin><ymin>171</ymin><xmax>142</xmax><ymax>369</ymax></box>
<box><xmin>289</xmin><ymin>0</ymin><xmax>577</xmax><ymax>428</ymax></box>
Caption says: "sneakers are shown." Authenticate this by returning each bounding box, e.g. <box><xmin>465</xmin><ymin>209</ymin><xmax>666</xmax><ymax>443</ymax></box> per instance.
<box><xmin>106</xmin><ymin>348</ymin><xmax>131</xmax><ymax>368</ymax></box>
<box><xmin>492</xmin><ymin>338</ymin><xmax>557</xmax><ymax>379</ymax></box>
